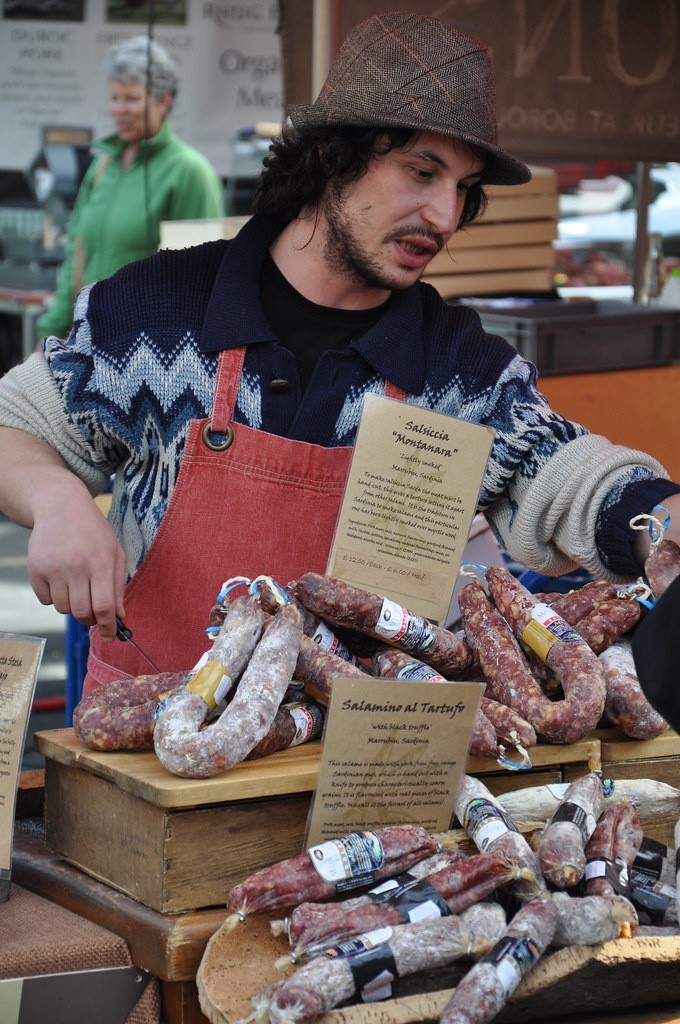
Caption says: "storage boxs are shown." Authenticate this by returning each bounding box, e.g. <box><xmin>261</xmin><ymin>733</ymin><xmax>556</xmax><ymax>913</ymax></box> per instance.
<box><xmin>32</xmin><ymin>726</ymin><xmax>597</xmax><ymax>914</ymax></box>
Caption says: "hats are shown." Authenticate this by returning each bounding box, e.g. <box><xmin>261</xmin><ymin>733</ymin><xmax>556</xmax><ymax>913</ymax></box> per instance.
<box><xmin>285</xmin><ymin>11</ymin><xmax>533</xmax><ymax>186</ymax></box>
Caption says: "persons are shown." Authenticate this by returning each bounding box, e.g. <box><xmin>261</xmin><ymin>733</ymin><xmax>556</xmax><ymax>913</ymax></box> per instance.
<box><xmin>39</xmin><ymin>36</ymin><xmax>224</xmax><ymax>354</ymax></box>
<box><xmin>0</xmin><ymin>13</ymin><xmax>680</xmax><ymax>730</ymax></box>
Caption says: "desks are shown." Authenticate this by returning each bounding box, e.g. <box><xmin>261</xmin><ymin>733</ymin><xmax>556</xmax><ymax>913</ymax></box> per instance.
<box><xmin>13</xmin><ymin>823</ymin><xmax>227</xmax><ymax>1024</ymax></box>
